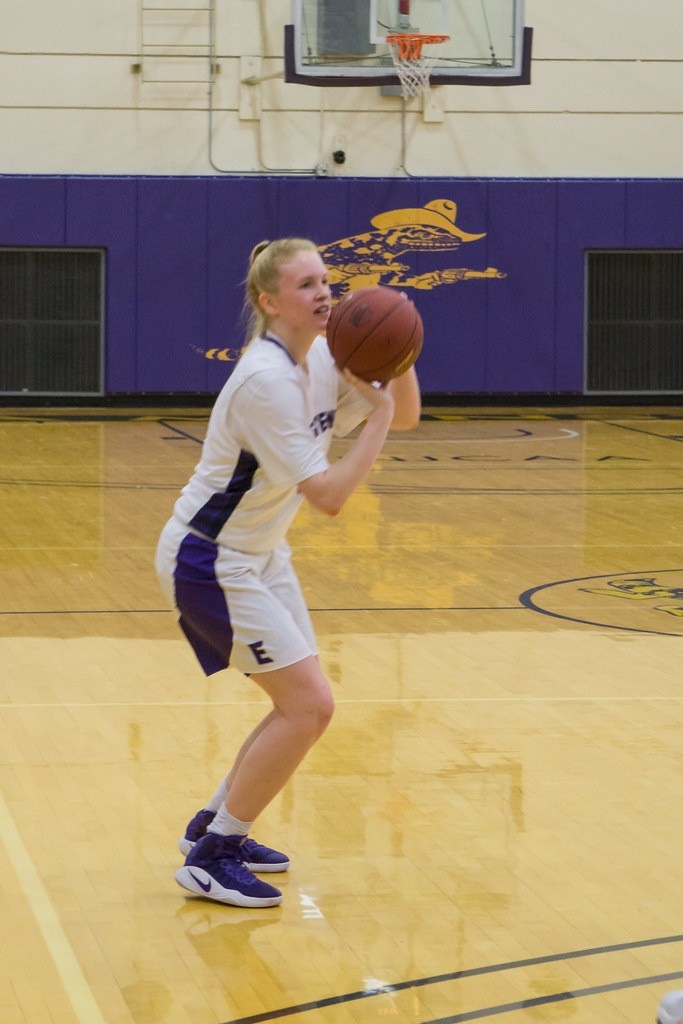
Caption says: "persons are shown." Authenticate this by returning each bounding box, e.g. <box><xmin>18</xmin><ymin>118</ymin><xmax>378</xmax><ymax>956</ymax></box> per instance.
<box><xmin>155</xmin><ymin>238</ymin><xmax>423</xmax><ymax>908</ymax></box>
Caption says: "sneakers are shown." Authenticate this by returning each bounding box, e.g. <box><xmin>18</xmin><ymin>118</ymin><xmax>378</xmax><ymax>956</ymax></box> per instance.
<box><xmin>177</xmin><ymin>809</ymin><xmax>290</xmax><ymax>873</ymax></box>
<box><xmin>175</xmin><ymin>832</ymin><xmax>284</xmax><ymax>908</ymax></box>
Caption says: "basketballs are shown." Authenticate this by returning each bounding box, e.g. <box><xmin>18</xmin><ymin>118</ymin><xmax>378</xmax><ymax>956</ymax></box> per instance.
<box><xmin>326</xmin><ymin>286</ymin><xmax>425</xmax><ymax>382</ymax></box>
